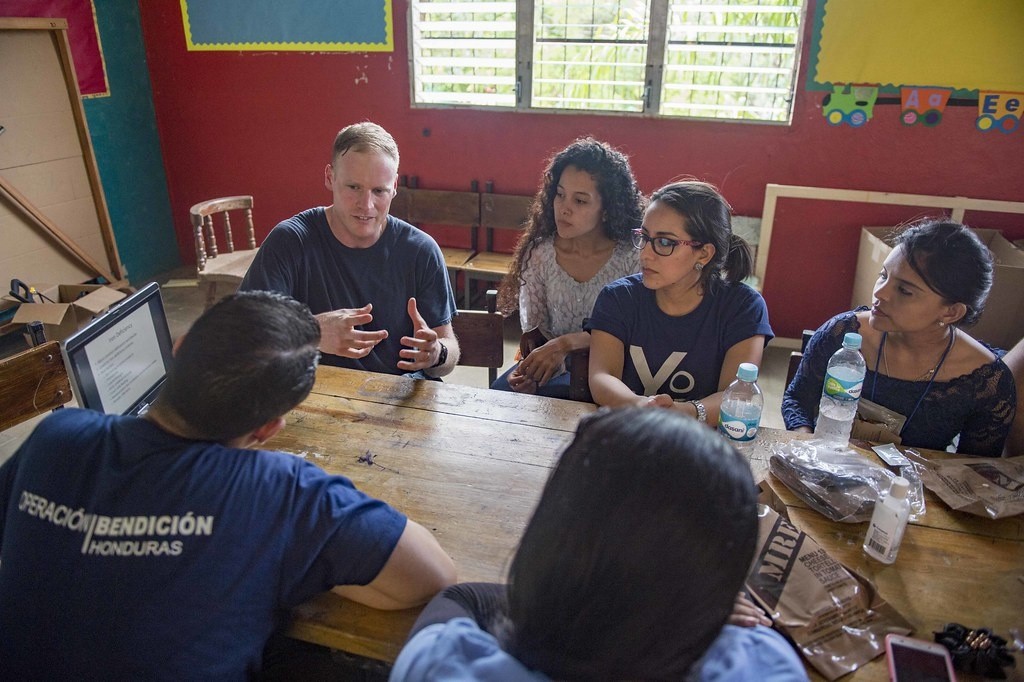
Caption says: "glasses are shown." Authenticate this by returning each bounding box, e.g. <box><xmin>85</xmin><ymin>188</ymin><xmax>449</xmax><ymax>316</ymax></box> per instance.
<box><xmin>631</xmin><ymin>228</ymin><xmax>707</xmax><ymax>256</ymax></box>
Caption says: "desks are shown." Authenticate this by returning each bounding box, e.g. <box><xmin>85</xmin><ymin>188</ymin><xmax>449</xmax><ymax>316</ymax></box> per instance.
<box><xmin>248</xmin><ymin>366</ymin><xmax>1023</xmax><ymax>682</ymax></box>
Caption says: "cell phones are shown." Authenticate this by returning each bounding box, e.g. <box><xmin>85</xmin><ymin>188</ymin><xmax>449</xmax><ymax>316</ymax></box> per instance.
<box><xmin>885</xmin><ymin>634</ymin><xmax>956</xmax><ymax>682</ymax></box>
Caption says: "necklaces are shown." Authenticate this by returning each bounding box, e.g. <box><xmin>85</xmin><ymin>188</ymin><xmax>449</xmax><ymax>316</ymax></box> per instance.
<box><xmin>883</xmin><ymin>333</ymin><xmax>955</xmax><ymax>382</ymax></box>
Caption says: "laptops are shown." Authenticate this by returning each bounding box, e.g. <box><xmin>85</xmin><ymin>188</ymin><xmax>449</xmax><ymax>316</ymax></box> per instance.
<box><xmin>60</xmin><ymin>281</ymin><xmax>176</xmax><ymax>418</ymax></box>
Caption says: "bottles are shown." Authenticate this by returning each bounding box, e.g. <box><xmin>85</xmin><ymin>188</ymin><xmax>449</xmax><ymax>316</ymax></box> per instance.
<box><xmin>863</xmin><ymin>477</ymin><xmax>911</xmax><ymax>564</ymax></box>
<box><xmin>814</xmin><ymin>332</ymin><xmax>866</xmax><ymax>449</ymax></box>
<box><xmin>715</xmin><ymin>362</ymin><xmax>764</xmax><ymax>469</ymax></box>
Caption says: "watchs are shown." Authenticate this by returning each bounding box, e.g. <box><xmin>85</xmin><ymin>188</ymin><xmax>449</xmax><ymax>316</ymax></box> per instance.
<box><xmin>687</xmin><ymin>400</ymin><xmax>707</xmax><ymax>423</ymax></box>
<box><xmin>430</xmin><ymin>340</ymin><xmax>448</xmax><ymax>369</ymax></box>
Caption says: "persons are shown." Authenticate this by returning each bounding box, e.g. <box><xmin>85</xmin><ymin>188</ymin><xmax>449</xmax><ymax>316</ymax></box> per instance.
<box><xmin>583</xmin><ymin>181</ymin><xmax>774</xmax><ymax>428</ymax></box>
<box><xmin>780</xmin><ymin>217</ymin><xmax>1017</xmax><ymax>458</ymax></box>
<box><xmin>0</xmin><ymin>291</ymin><xmax>459</xmax><ymax>682</ymax></box>
<box><xmin>389</xmin><ymin>405</ymin><xmax>810</xmax><ymax>682</ymax></box>
<box><xmin>490</xmin><ymin>137</ymin><xmax>648</xmax><ymax>404</ymax></box>
<box><xmin>235</xmin><ymin>122</ymin><xmax>461</xmax><ymax>382</ymax></box>
<box><xmin>1002</xmin><ymin>338</ymin><xmax>1024</xmax><ymax>459</ymax></box>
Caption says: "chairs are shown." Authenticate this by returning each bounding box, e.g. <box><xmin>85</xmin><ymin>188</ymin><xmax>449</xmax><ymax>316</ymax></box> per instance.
<box><xmin>191</xmin><ymin>195</ymin><xmax>260</xmax><ymax>310</ymax></box>
<box><xmin>462</xmin><ymin>181</ymin><xmax>537</xmax><ymax>310</ymax></box>
<box><xmin>406</xmin><ymin>175</ymin><xmax>479</xmax><ymax>305</ymax></box>
<box><xmin>388</xmin><ymin>175</ymin><xmax>407</xmax><ymax>221</ymax></box>
<box><xmin>720</xmin><ymin>216</ymin><xmax>763</xmax><ymax>291</ymax></box>
<box><xmin>453</xmin><ymin>289</ymin><xmax>504</xmax><ymax>388</ymax></box>
<box><xmin>0</xmin><ymin>320</ymin><xmax>73</xmax><ymax>432</ymax></box>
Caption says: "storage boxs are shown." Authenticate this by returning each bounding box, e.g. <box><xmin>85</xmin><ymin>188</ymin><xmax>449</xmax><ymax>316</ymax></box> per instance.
<box><xmin>849</xmin><ymin>228</ymin><xmax>1024</xmax><ymax>351</ymax></box>
<box><xmin>0</xmin><ymin>282</ymin><xmax>127</xmax><ymax>343</ymax></box>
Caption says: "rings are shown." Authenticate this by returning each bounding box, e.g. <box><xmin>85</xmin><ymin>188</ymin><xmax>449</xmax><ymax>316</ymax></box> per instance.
<box><xmin>425</xmin><ymin>351</ymin><xmax>430</xmax><ymax>362</ymax></box>
